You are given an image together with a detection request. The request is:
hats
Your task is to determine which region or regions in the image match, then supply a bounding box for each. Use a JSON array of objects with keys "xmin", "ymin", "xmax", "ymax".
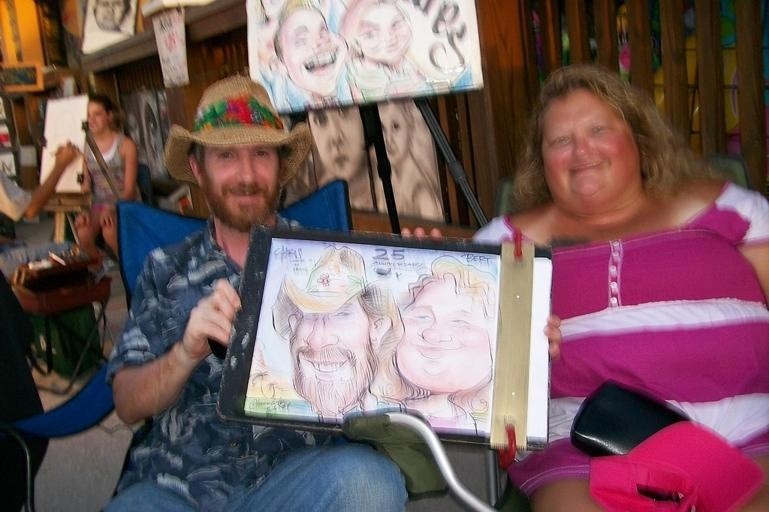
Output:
[{"xmin": 163, "ymin": 75, "xmax": 312, "ymax": 189}]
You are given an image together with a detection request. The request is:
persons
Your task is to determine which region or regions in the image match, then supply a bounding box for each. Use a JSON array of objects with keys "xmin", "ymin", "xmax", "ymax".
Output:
[
  {"xmin": 93, "ymin": 0, "xmax": 130, "ymax": 36},
  {"xmin": 97, "ymin": 72, "xmax": 562, "ymax": 512},
  {"xmin": 299, "ymin": 104, "xmax": 375, "ymax": 209},
  {"xmin": 391, "ymin": 253, "xmax": 495, "ymax": 429},
  {"xmin": 140, "ymin": 97, "xmax": 164, "ymax": 181},
  {"xmin": 270, "ymin": 244, "xmax": 406, "ymax": 419},
  {"xmin": 0, "ymin": 139, "xmax": 78, "ymax": 286},
  {"xmin": 336, "ymin": 0, "xmax": 428, "ymax": 80},
  {"xmin": 158, "ymin": 88, "xmax": 172, "ymax": 140},
  {"xmin": 74, "ymin": 92, "xmax": 139, "ymax": 262},
  {"xmin": 373, "ymin": 97, "xmax": 444, "ymax": 220},
  {"xmin": 398, "ymin": 63, "xmax": 768, "ymax": 511},
  {"xmin": 266, "ymin": 4, "xmax": 358, "ymax": 108},
  {"xmin": 121, "ymin": 99, "xmax": 146, "ymax": 164}
]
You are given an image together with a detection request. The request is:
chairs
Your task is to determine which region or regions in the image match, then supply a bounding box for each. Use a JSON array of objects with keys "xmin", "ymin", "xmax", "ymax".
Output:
[
  {"xmin": 96, "ymin": 163, "xmax": 157, "ymax": 266},
  {"xmin": 114, "ymin": 176, "xmax": 354, "ymax": 317}
]
